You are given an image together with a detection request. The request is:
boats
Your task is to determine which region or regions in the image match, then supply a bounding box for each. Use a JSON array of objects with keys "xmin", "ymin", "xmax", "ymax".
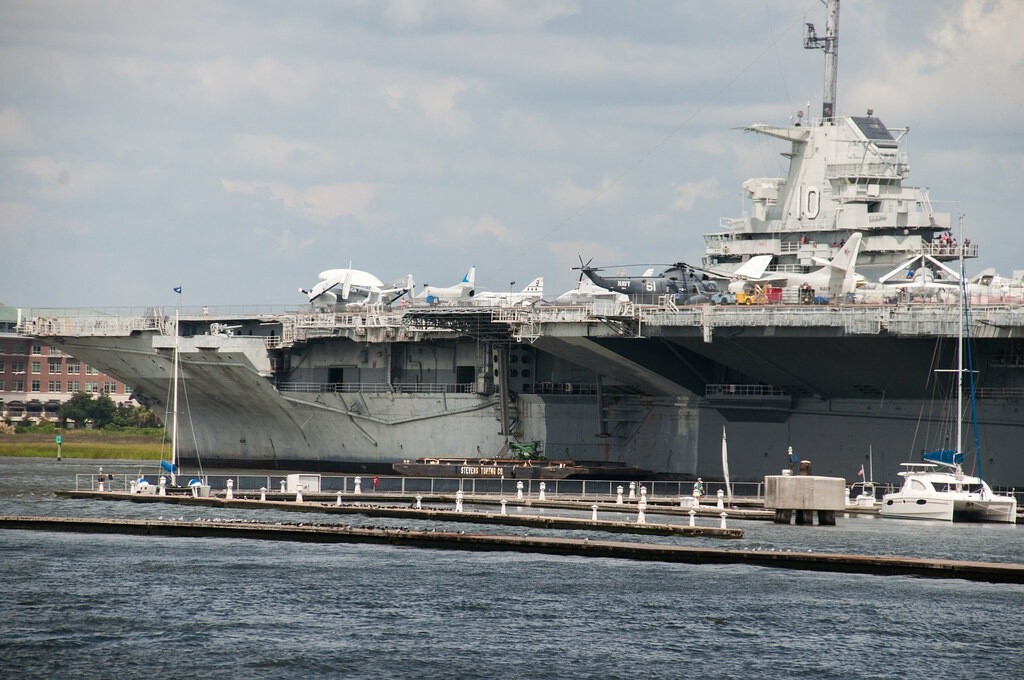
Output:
[{"xmin": 392, "ymin": 457, "xmax": 659, "ymax": 483}]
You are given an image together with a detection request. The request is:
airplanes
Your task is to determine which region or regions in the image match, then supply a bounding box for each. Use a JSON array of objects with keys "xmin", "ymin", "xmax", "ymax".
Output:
[{"xmin": 297, "ymin": 228, "xmax": 1023, "ymax": 313}]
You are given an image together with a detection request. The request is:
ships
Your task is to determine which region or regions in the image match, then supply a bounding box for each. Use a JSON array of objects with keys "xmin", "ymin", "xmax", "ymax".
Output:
[{"xmin": 11, "ymin": 2, "xmax": 1024, "ymax": 507}]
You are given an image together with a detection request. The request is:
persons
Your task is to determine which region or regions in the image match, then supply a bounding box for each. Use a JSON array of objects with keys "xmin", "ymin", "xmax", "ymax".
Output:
[
  {"xmin": 202, "ymin": 305, "xmax": 208, "ymax": 317},
  {"xmin": 935, "ymin": 231, "xmax": 957, "ymax": 254},
  {"xmin": 962, "ymin": 238, "xmax": 971, "ymax": 254},
  {"xmin": 400, "ymin": 296, "xmax": 411, "ymax": 311},
  {"xmin": 832, "ymin": 239, "xmax": 845, "ymax": 247}
]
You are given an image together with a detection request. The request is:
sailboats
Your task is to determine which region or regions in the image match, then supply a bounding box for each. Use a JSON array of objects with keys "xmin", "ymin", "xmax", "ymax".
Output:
[
  {"xmin": 879, "ymin": 211, "xmax": 1017, "ymax": 524},
  {"xmin": 129, "ymin": 307, "xmax": 221, "ymax": 496}
]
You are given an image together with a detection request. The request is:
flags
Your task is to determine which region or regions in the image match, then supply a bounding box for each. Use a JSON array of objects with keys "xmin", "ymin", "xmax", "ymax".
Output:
[{"xmin": 173, "ymin": 287, "xmax": 182, "ymax": 294}]
